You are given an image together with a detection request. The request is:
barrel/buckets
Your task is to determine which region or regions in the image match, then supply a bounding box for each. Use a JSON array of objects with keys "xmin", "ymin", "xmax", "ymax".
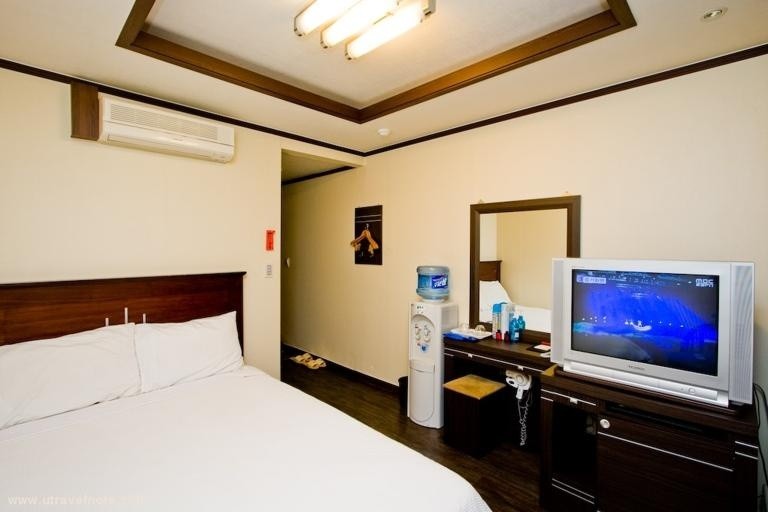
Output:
[{"xmin": 416, "ymin": 265, "xmax": 449, "ymax": 300}]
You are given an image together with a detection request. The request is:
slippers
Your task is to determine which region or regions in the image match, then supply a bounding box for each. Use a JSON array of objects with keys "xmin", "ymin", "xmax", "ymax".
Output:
[{"xmin": 290, "ymin": 353, "xmax": 326, "ymax": 369}]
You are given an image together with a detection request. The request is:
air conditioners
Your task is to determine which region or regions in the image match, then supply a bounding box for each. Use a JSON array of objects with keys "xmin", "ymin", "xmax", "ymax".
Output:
[{"xmin": 97, "ymin": 91, "xmax": 235, "ymax": 165}]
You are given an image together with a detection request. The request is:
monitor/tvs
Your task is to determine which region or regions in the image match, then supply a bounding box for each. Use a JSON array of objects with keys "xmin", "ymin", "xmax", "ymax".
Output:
[{"xmin": 563, "ymin": 257, "xmax": 731, "ymax": 408}]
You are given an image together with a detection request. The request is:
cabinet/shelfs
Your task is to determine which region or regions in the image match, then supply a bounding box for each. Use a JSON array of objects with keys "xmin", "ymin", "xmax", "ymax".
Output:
[{"xmin": 538, "ymin": 363, "xmax": 759, "ymax": 512}]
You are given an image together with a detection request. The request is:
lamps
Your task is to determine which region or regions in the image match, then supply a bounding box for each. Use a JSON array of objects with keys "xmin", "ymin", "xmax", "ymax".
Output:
[{"xmin": 293, "ymin": 0, "xmax": 436, "ymax": 61}]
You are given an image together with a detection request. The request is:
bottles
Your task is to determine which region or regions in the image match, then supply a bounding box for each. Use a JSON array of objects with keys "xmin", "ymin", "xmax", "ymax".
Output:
[
  {"xmin": 517, "ymin": 315, "xmax": 525, "ymax": 330},
  {"xmin": 491, "ymin": 303, "xmax": 520, "ymax": 345}
]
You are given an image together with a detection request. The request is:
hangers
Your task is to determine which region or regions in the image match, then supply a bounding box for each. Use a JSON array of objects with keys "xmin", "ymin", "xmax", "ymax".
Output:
[{"xmin": 350, "ymin": 224, "xmax": 379, "ymax": 255}]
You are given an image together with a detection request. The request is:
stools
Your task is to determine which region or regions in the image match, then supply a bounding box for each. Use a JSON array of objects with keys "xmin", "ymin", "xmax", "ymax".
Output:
[{"xmin": 441, "ymin": 374, "xmax": 508, "ymax": 459}]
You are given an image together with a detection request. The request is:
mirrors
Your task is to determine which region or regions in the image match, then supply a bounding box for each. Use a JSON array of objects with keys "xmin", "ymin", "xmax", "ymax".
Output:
[{"xmin": 468, "ymin": 195, "xmax": 581, "ymax": 344}]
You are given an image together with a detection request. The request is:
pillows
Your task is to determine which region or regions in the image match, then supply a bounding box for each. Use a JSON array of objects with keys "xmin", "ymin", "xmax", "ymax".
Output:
[
  {"xmin": 479, "ymin": 280, "xmax": 513, "ymax": 324},
  {"xmin": 0, "ymin": 321, "xmax": 142, "ymax": 430},
  {"xmin": 134, "ymin": 310, "xmax": 246, "ymax": 392}
]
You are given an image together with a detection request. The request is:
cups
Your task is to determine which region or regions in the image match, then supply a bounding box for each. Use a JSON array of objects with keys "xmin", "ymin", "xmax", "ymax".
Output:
[{"xmin": 474, "ymin": 325, "xmax": 486, "ymax": 337}]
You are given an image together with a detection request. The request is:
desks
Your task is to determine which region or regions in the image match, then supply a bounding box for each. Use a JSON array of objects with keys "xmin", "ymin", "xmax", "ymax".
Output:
[{"xmin": 443, "ymin": 328, "xmax": 555, "ymax": 454}]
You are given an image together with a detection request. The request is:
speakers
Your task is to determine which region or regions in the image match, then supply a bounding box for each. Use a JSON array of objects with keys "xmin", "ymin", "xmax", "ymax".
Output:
[
  {"xmin": 729, "ymin": 261, "xmax": 755, "ymax": 405},
  {"xmin": 550, "ymin": 257, "xmax": 564, "ymax": 365}
]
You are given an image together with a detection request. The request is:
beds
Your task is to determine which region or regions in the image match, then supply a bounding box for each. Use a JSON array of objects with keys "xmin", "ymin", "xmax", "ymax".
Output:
[
  {"xmin": 479, "ymin": 260, "xmax": 551, "ymax": 334},
  {"xmin": 0, "ymin": 270, "xmax": 496, "ymax": 512}
]
